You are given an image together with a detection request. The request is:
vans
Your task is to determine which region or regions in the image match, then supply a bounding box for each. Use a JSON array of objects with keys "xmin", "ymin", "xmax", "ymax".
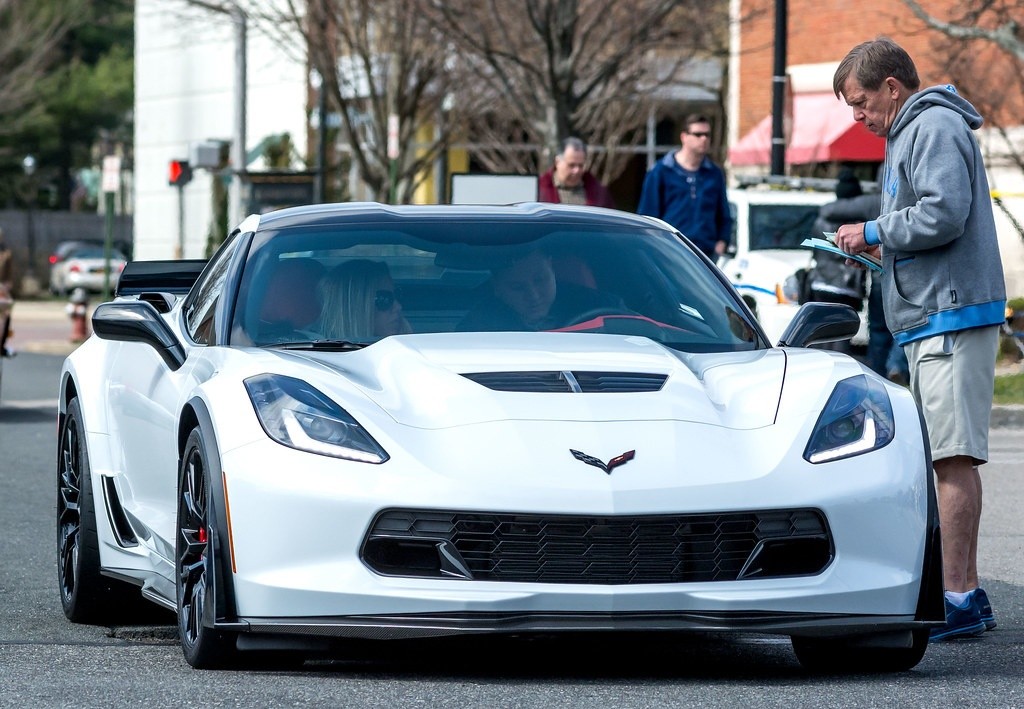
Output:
[{"xmin": 711, "ymin": 182, "xmax": 875, "ymax": 351}]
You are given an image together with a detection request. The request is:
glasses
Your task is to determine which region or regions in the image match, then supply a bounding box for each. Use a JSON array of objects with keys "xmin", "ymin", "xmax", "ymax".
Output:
[
  {"xmin": 688, "ymin": 132, "xmax": 711, "ymax": 137},
  {"xmin": 376, "ymin": 286, "xmax": 402, "ymax": 312}
]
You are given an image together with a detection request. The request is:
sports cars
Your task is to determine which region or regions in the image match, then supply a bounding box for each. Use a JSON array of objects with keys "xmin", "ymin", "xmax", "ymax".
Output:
[{"xmin": 54, "ymin": 200, "xmax": 950, "ymax": 675}]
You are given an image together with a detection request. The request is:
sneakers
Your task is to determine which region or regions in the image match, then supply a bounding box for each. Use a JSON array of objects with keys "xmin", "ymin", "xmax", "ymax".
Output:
[{"xmin": 929, "ymin": 588, "xmax": 997, "ymax": 642}]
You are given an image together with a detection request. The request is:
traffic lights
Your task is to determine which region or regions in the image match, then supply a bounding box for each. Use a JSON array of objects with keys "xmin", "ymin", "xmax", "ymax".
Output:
[{"xmin": 170, "ymin": 160, "xmax": 193, "ymax": 189}]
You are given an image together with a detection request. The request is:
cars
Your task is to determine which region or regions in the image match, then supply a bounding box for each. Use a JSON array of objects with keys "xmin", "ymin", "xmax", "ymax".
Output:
[{"xmin": 47, "ymin": 243, "xmax": 129, "ymax": 294}]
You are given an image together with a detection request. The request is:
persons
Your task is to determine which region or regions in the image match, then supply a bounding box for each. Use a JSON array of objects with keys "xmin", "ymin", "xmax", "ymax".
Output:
[
  {"xmin": 303, "ymin": 259, "xmax": 411, "ymax": 345},
  {"xmin": 832, "ymin": 40, "xmax": 1006, "ymax": 640},
  {"xmin": 0, "ymin": 242, "xmax": 17, "ymax": 355},
  {"xmin": 537, "ymin": 137, "xmax": 613, "ymax": 206},
  {"xmin": 639, "ymin": 114, "xmax": 730, "ymax": 261},
  {"xmin": 456, "ymin": 247, "xmax": 626, "ymax": 339}
]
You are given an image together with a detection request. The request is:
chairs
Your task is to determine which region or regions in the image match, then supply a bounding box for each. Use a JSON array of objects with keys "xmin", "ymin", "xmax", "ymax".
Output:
[{"xmin": 269, "ymin": 259, "xmax": 327, "ymax": 332}]
